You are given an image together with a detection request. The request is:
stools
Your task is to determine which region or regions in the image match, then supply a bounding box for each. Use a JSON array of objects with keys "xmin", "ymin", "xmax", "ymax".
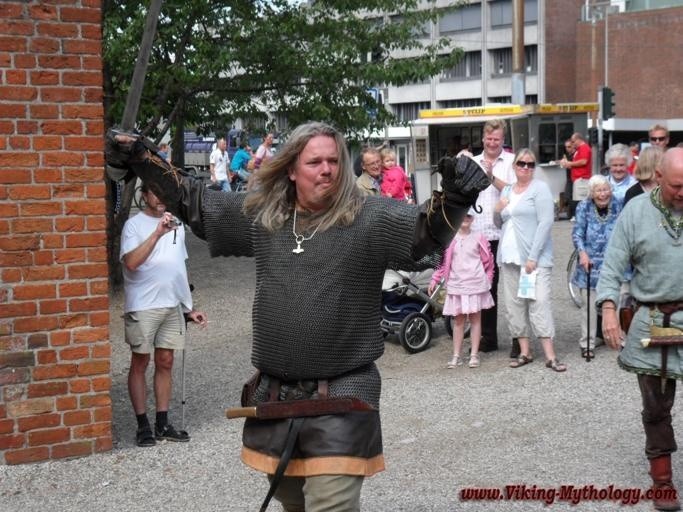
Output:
[{"xmin": 230, "ymin": 172, "xmax": 247, "ymax": 191}]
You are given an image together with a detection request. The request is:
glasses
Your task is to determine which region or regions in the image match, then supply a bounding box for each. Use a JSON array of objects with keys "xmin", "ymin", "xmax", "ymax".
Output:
[
  {"xmin": 649, "ymin": 136, "xmax": 667, "ymax": 142},
  {"xmin": 517, "ymin": 160, "xmax": 536, "ymax": 169}
]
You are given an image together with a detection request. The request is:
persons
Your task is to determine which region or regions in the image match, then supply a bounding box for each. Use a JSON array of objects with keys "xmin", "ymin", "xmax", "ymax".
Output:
[
  {"xmin": 560, "ymin": 124, "xmax": 683, "ymax": 361},
  {"xmin": 152, "ymin": 128, "xmax": 278, "ymax": 196},
  {"xmin": 596, "ymin": 148, "xmax": 683, "ymax": 512},
  {"xmin": 356, "ymin": 116, "xmax": 568, "ymax": 373},
  {"xmin": 118, "ymin": 180, "xmax": 205, "ymax": 449},
  {"xmin": 106, "ymin": 122, "xmax": 490, "ymax": 511}
]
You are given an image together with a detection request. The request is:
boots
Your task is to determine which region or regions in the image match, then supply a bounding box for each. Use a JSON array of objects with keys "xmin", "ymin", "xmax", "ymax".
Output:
[{"xmin": 649, "ymin": 454, "xmax": 680, "ymax": 511}]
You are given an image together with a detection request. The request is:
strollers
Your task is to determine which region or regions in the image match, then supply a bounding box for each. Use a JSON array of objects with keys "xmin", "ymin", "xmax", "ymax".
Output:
[{"xmin": 380, "ymin": 267, "xmax": 469, "ymax": 353}]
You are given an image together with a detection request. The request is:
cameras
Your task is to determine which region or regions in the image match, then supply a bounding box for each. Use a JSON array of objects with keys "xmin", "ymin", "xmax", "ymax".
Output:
[{"xmin": 168, "ymin": 216, "xmax": 183, "ymax": 227}]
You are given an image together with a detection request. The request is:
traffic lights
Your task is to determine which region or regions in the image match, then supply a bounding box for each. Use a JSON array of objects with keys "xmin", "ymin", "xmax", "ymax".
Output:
[{"xmin": 601, "ymin": 88, "xmax": 616, "ymax": 120}]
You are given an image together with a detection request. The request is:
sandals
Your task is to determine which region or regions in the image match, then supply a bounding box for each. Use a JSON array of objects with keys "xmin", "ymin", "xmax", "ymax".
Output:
[
  {"xmin": 448, "ymin": 343, "xmax": 595, "ymax": 372},
  {"xmin": 136, "ymin": 426, "xmax": 155, "ymax": 447},
  {"xmin": 154, "ymin": 423, "xmax": 190, "ymax": 442}
]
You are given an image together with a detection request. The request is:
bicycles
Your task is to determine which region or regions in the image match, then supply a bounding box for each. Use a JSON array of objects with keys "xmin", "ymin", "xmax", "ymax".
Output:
[{"xmin": 566, "ymin": 248, "xmax": 583, "ymax": 309}]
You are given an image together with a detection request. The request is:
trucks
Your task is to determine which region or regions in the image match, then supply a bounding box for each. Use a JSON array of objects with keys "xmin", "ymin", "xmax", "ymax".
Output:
[{"xmin": 183, "ymin": 130, "xmax": 242, "ymax": 180}]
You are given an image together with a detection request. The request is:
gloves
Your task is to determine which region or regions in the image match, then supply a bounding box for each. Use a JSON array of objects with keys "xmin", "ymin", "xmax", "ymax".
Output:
[
  {"xmin": 104, "ymin": 127, "xmax": 208, "ymax": 242},
  {"xmin": 410, "ymin": 154, "xmax": 491, "ymax": 264}
]
